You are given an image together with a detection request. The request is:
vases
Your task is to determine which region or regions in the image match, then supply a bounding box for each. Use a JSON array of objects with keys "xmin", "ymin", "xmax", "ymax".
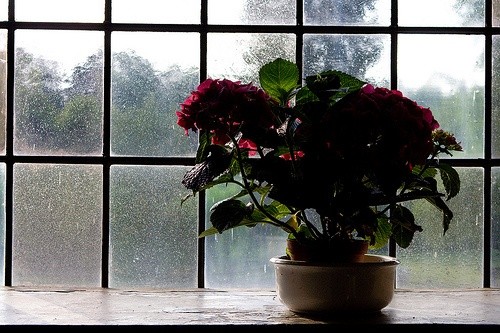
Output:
[{"xmin": 269, "ymin": 239, "xmax": 402, "ymax": 321}]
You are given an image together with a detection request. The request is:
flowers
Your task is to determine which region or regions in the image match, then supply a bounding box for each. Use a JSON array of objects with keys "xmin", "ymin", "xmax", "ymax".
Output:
[{"xmin": 174, "ymin": 56, "xmax": 465, "ymax": 239}]
